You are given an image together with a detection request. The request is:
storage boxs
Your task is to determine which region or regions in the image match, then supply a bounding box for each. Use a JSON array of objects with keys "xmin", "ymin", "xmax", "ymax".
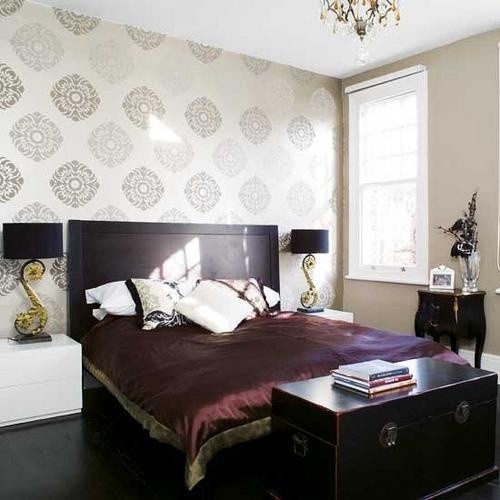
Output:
[{"xmin": 272, "ymin": 356, "xmax": 499, "ymax": 500}]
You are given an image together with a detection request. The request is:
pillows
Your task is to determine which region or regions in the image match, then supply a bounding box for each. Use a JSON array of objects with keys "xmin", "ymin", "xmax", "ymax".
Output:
[
  {"xmin": 178, "ymin": 282, "xmax": 255, "ymax": 335},
  {"xmin": 84, "ymin": 278, "xmax": 279, "ymax": 321},
  {"xmin": 126, "ymin": 277, "xmax": 194, "ymax": 332},
  {"xmin": 203, "ymin": 278, "xmax": 268, "ymax": 322}
]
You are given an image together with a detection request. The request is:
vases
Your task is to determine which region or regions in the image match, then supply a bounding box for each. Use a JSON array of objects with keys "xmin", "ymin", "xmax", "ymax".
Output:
[{"xmin": 455, "ymin": 250, "xmax": 480, "ymax": 294}]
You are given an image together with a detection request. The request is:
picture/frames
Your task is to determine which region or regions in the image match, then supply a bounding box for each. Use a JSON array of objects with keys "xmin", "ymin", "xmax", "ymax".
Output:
[{"xmin": 430, "ymin": 266, "xmax": 454, "ymax": 289}]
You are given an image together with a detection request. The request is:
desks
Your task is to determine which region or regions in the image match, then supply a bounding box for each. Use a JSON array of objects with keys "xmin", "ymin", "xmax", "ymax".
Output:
[{"xmin": 414, "ymin": 288, "xmax": 487, "ymax": 368}]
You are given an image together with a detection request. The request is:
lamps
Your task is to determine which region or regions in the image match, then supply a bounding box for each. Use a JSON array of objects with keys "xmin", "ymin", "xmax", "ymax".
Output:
[
  {"xmin": 291, "ymin": 230, "xmax": 330, "ymax": 312},
  {"xmin": 321, "ymin": 0, "xmax": 399, "ymax": 42},
  {"xmin": 4, "ymin": 224, "xmax": 62, "ymax": 344}
]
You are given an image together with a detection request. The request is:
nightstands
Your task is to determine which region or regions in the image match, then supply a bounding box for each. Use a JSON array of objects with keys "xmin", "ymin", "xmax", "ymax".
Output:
[
  {"xmin": 292, "ymin": 309, "xmax": 354, "ymax": 324},
  {"xmin": 0, "ymin": 336, "xmax": 82, "ymax": 431}
]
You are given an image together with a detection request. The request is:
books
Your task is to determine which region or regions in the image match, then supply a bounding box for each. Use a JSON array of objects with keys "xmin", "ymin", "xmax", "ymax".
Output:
[{"xmin": 330, "ymin": 357, "xmax": 417, "ymax": 402}]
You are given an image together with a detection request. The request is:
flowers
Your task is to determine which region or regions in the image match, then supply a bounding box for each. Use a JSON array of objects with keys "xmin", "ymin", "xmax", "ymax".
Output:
[{"xmin": 437, "ymin": 190, "xmax": 480, "ymax": 279}]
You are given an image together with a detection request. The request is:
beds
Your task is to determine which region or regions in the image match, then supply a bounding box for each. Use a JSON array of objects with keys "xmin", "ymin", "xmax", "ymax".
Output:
[{"xmin": 68, "ymin": 220, "xmax": 474, "ymax": 500}]
